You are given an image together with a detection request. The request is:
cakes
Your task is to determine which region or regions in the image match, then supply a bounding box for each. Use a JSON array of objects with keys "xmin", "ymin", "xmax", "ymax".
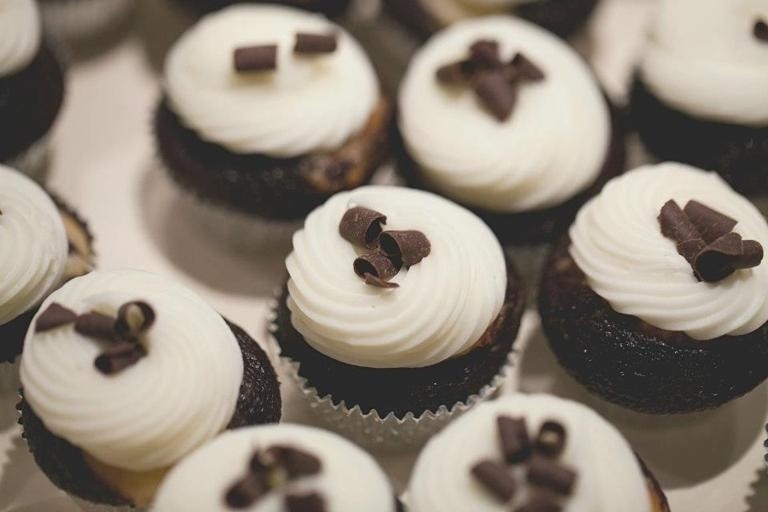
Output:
[{"xmin": 0, "ymin": 0, "xmax": 768, "ymax": 512}]
